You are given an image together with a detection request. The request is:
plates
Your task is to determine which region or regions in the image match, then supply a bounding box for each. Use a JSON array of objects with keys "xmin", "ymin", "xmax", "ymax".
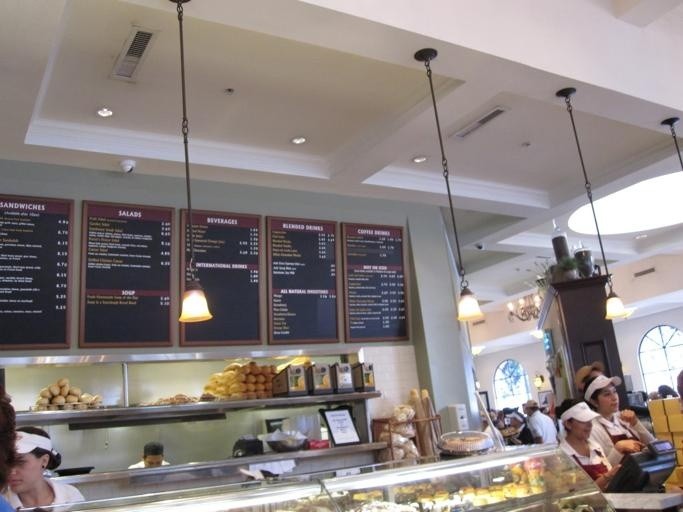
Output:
[{"xmin": 53, "ymin": 466, "xmax": 95, "ymax": 477}]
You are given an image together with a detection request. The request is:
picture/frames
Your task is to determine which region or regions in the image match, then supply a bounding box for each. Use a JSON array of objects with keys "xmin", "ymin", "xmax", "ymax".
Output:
[
  {"xmin": 318, "ymin": 404, "xmax": 361, "ymax": 446},
  {"xmin": 478, "ymin": 391, "xmax": 490, "ymax": 413}
]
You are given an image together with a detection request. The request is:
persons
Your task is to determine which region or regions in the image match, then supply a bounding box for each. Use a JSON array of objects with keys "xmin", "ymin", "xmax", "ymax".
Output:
[
  {"xmin": 482, "ymin": 360, "xmax": 683, "ymax": 493},
  {"xmin": 0, "ymin": 384, "xmax": 19, "ymax": 512},
  {"xmin": 2, "ymin": 426, "xmax": 85, "ymax": 512},
  {"xmin": 126, "ymin": 440, "xmax": 170, "ymax": 487}
]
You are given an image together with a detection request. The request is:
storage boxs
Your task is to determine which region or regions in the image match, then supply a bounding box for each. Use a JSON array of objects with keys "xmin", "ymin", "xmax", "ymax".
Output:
[
  {"xmin": 353, "ymin": 362, "xmax": 376, "ymax": 392},
  {"xmin": 330, "ymin": 362, "xmax": 355, "ymax": 395},
  {"xmin": 306, "ymin": 362, "xmax": 334, "ymax": 395},
  {"xmin": 272, "ymin": 364, "xmax": 308, "ymax": 397}
]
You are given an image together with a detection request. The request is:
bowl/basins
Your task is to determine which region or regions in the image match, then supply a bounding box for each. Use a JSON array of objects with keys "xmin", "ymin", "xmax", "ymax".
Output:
[{"xmin": 265, "ymin": 438, "xmax": 308, "ymax": 451}]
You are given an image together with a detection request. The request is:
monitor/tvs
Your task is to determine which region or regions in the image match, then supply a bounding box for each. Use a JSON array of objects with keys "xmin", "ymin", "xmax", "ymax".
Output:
[{"xmin": 606, "ymin": 447, "xmax": 679, "ymax": 492}]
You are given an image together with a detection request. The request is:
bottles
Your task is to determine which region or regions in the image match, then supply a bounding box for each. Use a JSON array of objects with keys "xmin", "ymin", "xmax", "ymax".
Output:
[{"xmin": 550, "ymin": 217, "xmax": 594, "ymax": 277}]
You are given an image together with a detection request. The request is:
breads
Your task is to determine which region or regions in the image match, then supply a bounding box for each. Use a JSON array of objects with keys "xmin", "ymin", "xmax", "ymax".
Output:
[
  {"xmin": 140, "ymin": 361, "xmax": 277, "ymax": 406},
  {"xmin": 34, "ymin": 378, "xmax": 100, "ymax": 411},
  {"xmin": 417, "ymin": 481, "xmax": 529, "ymax": 511},
  {"xmin": 352, "ymin": 483, "xmax": 431, "ymax": 502},
  {"xmin": 377, "ymin": 389, "xmax": 429, "ymax": 462}
]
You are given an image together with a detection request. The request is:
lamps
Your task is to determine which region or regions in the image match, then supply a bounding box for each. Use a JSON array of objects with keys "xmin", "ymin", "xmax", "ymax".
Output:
[
  {"xmin": 533, "ymin": 373, "xmax": 545, "ymax": 388},
  {"xmin": 555, "ymin": 87, "xmax": 629, "ymax": 320},
  {"xmin": 168, "ymin": 0, "xmax": 214, "ymax": 324},
  {"xmin": 506, "ymin": 294, "xmax": 545, "ymax": 322},
  {"xmin": 413, "ymin": 48, "xmax": 486, "ymax": 322}
]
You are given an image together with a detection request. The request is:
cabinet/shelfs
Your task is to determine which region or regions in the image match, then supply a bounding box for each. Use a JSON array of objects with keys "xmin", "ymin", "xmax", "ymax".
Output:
[{"xmin": 15, "ymin": 389, "xmax": 388, "ymax": 485}]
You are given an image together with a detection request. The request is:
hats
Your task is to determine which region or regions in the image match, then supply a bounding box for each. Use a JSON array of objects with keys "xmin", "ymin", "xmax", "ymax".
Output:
[
  {"xmin": 13, "ymin": 429, "xmax": 58, "ymax": 458},
  {"xmin": 525, "ymin": 399, "xmax": 540, "ymax": 409},
  {"xmin": 573, "ymin": 360, "xmax": 606, "ymax": 392},
  {"xmin": 560, "ymin": 399, "xmax": 603, "ymax": 423},
  {"xmin": 582, "ymin": 374, "xmax": 624, "ymax": 403}
]
